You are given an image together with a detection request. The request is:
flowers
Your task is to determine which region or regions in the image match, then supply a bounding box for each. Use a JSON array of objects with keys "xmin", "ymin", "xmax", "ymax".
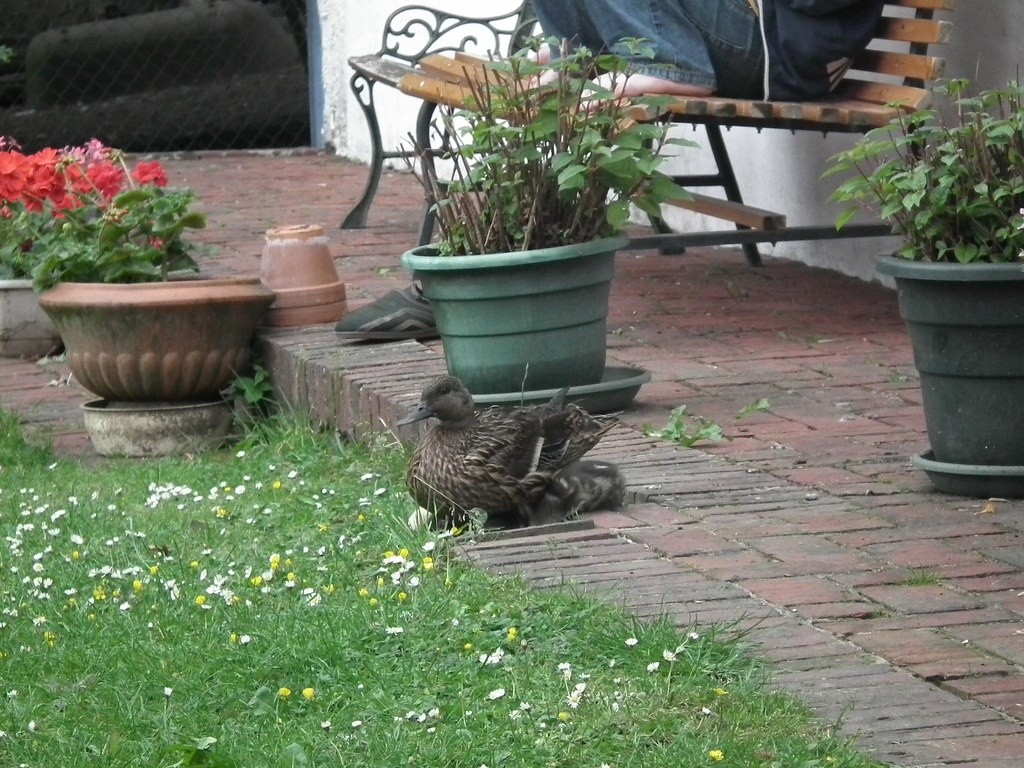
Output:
[{"xmin": 0, "ymin": 134, "xmax": 220, "ymax": 294}]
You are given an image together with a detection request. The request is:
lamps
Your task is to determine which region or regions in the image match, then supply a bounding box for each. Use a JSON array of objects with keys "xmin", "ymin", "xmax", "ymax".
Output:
[{"xmin": 0, "ymin": 279, "xmax": 64, "ymax": 358}]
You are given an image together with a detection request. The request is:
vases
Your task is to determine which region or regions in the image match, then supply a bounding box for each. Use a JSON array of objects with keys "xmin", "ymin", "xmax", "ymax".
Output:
[
  {"xmin": 44, "ymin": 275, "xmax": 278, "ymax": 407},
  {"xmin": 257, "ymin": 220, "xmax": 347, "ymax": 324}
]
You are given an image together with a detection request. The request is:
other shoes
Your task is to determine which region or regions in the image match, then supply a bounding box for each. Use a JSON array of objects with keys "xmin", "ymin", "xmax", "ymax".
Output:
[{"xmin": 335, "ymin": 284, "xmax": 441, "ymax": 339}]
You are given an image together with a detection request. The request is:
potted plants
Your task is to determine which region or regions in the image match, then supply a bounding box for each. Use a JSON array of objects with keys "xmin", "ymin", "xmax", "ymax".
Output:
[
  {"xmin": 822, "ymin": 53, "xmax": 1024, "ymax": 466},
  {"xmin": 398, "ymin": 33, "xmax": 702, "ymax": 394}
]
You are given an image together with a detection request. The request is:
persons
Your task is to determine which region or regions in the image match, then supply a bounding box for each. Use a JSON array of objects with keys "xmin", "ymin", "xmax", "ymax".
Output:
[{"xmin": 524, "ymin": 0, "xmax": 884, "ymax": 110}]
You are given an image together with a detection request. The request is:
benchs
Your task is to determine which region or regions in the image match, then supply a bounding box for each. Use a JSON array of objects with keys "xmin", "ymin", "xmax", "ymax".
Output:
[{"xmin": 341, "ymin": 0, "xmax": 959, "ymax": 283}]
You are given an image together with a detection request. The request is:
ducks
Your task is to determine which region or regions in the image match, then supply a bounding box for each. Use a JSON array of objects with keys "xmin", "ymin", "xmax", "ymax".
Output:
[{"xmin": 397, "ymin": 373, "xmax": 628, "ymax": 521}]
